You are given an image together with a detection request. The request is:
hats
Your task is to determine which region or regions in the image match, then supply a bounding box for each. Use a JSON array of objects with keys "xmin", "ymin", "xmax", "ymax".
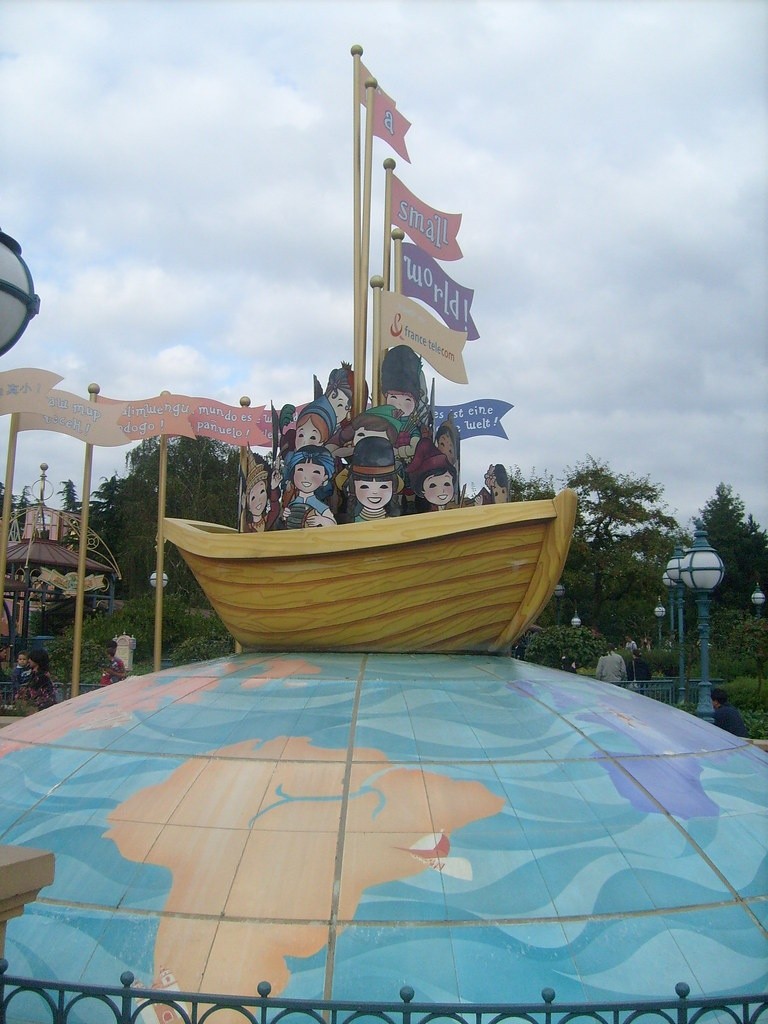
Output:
[
  {"xmin": 633, "ymin": 649, "xmax": 642, "ymax": 657},
  {"xmin": 107, "ymin": 647, "xmax": 115, "ymax": 657},
  {"xmin": 0, "ymin": 646, "xmax": 4, "ymax": 652},
  {"xmin": 28, "ymin": 648, "xmax": 50, "ymax": 667}
]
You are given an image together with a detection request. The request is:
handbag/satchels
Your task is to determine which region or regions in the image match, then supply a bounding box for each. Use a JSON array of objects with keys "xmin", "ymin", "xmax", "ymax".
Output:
[{"xmin": 628, "ymin": 680, "xmax": 638, "ymax": 691}]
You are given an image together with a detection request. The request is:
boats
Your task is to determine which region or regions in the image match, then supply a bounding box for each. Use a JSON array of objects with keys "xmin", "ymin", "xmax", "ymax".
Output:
[{"xmin": 159, "ymin": 42, "xmax": 581, "ymax": 653}]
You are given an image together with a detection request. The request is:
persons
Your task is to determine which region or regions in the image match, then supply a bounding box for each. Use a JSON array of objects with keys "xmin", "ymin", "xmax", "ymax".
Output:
[
  {"xmin": 711, "ymin": 688, "xmax": 749, "ymax": 738},
  {"xmin": 98, "ymin": 640, "xmax": 127, "ymax": 685},
  {"xmin": 596, "ymin": 631, "xmax": 672, "ymax": 695},
  {"xmin": 0, "ymin": 647, "xmax": 57, "ymax": 711}
]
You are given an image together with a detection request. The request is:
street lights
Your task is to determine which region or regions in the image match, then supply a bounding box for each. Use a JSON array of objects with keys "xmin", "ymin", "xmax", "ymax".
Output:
[
  {"xmin": 653, "ymin": 519, "xmax": 727, "ymax": 726},
  {"xmin": 554, "ymin": 584, "xmax": 581, "ymax": 629},
  {"xmin": 750, "ymin": 582, "xmax": 766, "ymax": 619}
]
